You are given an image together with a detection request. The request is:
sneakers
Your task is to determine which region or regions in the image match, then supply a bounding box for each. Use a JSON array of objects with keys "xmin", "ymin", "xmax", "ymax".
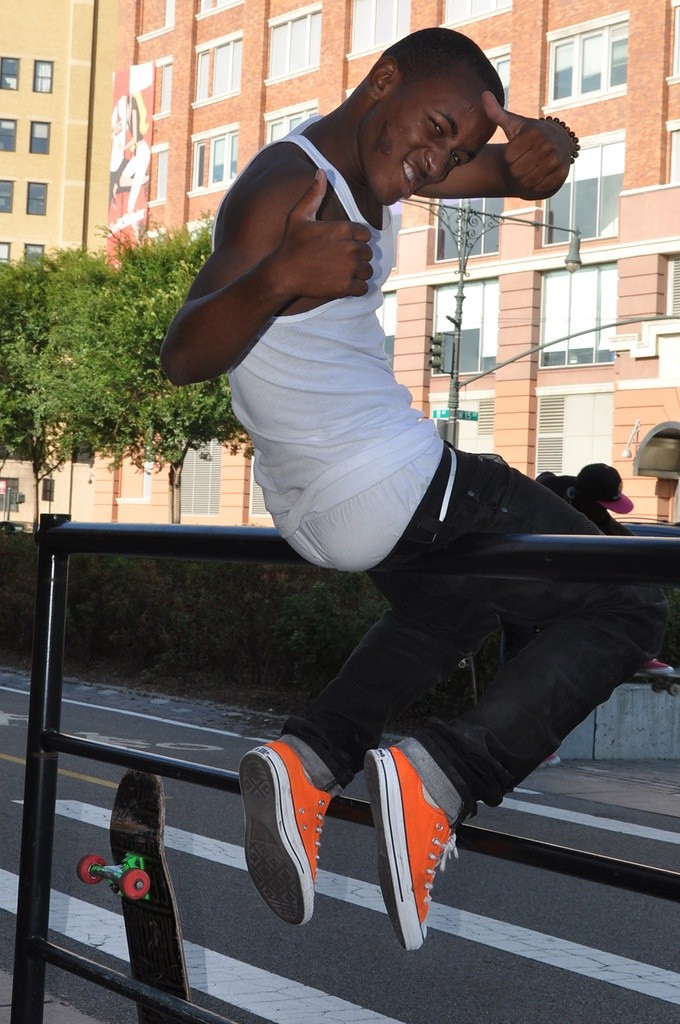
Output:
[
  {"xmin": 239, "ymin": 740, "xmax": 331, "ymax": 926},
  {"xmin": 636, "ymin": 658, "xmax": 675, "ymax": 674},
  {"xmin": 366, "ymin": 745, "xmax": 456, "ymax": 951}
]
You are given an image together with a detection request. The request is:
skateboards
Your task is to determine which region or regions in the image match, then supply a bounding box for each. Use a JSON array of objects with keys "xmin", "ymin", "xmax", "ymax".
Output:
[{"xmin": 73, "ymin": 768, "xmax": 194, "ymax": 1024}]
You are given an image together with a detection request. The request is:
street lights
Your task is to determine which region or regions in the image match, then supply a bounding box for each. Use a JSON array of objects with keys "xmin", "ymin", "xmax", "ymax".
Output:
[{"xmin": 399, "ymin": 194, "xmax": 582, "ymax": 450}]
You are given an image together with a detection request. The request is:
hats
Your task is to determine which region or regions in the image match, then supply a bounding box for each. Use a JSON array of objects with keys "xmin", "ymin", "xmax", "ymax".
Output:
[{"xmin": 578, "ymin": 463, "xmax": 633, "ymax": 514}]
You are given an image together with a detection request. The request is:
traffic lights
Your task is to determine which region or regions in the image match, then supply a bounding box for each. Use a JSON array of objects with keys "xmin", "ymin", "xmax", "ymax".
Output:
[{"xmin": 428, "ymin": 333, "xmax": 445, "ymax": 370}]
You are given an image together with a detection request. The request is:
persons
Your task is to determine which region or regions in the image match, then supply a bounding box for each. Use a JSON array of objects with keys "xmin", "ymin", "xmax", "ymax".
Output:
[
  {"xmin": 534, "ymin": 464, "xmax": 674, "ymax": 675},
  {"xmin": 159, "ymin": 27, "xmax": 668, "ymax": 956}
]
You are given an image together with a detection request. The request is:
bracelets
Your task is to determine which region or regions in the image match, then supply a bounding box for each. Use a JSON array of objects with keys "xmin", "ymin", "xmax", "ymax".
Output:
[{"xmin": 539, "ymin": 116, "xmax": 582, "ymax": 164}]
